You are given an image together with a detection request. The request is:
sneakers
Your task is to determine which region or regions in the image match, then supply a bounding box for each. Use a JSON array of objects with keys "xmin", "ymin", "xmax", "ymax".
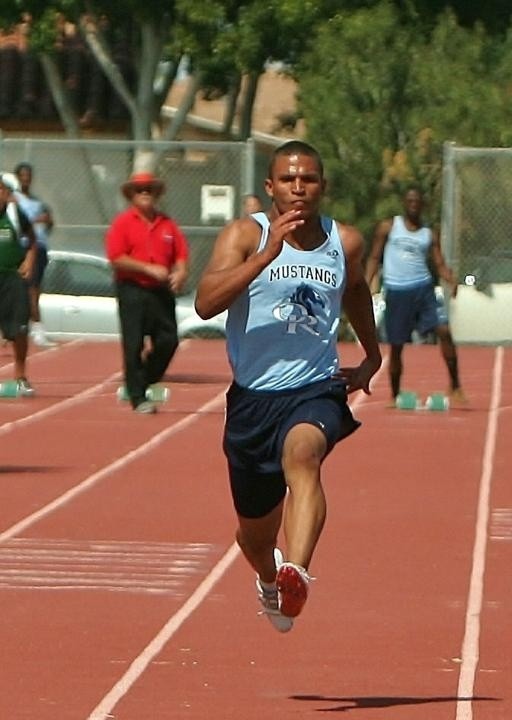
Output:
[
  {"xmin": 448, "ymin": 387, "xmax": 465, "ymax": 402},
  {"xmin": 16, "ymin": 377, "xmax": 34, "ymax": 394},
  {"xmin": 135, "ymin": 402, "xmax": 156, "ymax": 414},
  {"xmin": 255, "ymin": 548, "xmax": 316, "ymax": 634}
]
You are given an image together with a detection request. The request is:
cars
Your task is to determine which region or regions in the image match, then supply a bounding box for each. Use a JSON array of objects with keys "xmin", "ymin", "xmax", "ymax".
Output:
[{"xmin": 28, "ymin": 250, "xmax": 228, "ymax": 337}]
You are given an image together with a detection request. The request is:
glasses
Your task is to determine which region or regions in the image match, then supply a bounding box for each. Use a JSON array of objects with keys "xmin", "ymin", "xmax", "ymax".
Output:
[{"xmin": 135, "ymin": 186, "xmax": 152, "ymax": 193}]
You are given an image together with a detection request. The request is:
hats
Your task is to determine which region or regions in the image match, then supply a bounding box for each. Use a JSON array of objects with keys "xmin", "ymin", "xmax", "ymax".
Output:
[{"xmin": 121, "ymin": 172, "xmax": 162, "ymax": 197}]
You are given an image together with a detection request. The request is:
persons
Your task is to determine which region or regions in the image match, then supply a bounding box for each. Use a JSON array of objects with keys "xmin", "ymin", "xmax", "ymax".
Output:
[
  {"xmin": 194, "ymin": 143, "xmax": 383, "ymax": 633},
  {"xmin": 242, "ymin": 195, "xmax": 262, "ymax": 213},
  {"xmin": 105, "ymin": 174, "xmax": 189, "ymax": 415},
  {"xmin": 0, "ymin": 171, "xmax": 38, "ymax": 396},
  {"xmin": 14, "ymin": 163, "xmax": 59, "ymax": 348},
  {"xmin": 364, "ymin": 188, "xmax": 472, "ymax": 411}
]
